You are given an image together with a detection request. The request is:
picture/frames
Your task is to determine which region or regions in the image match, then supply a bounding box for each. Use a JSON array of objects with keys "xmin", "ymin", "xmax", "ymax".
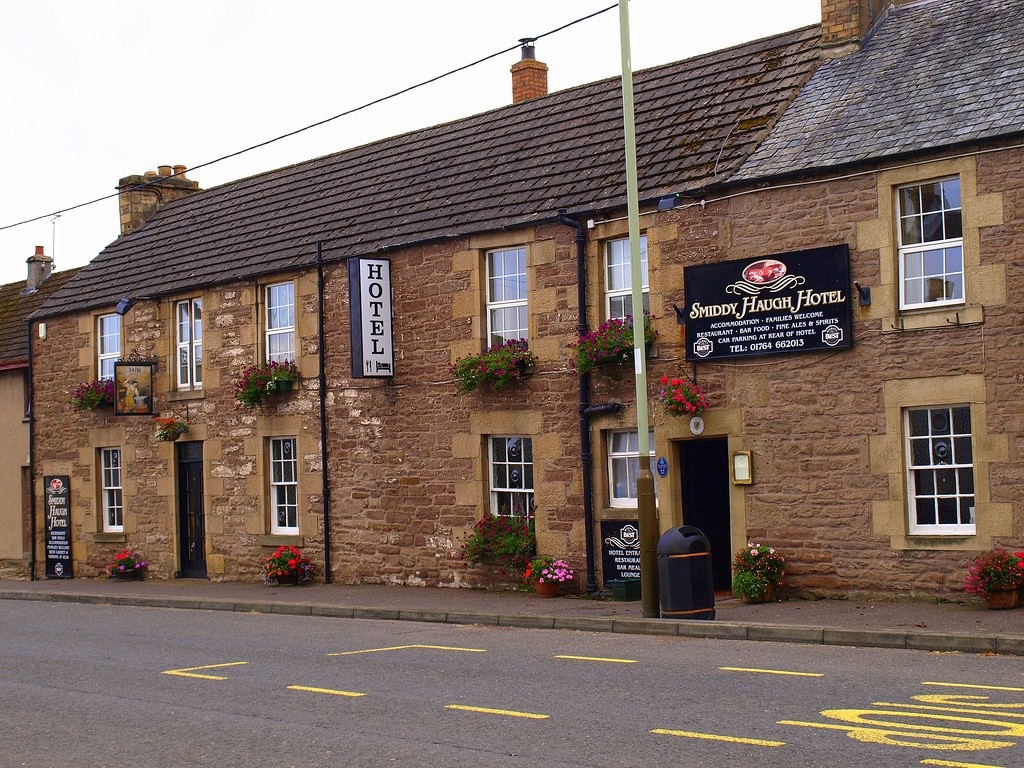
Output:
[
  {"xmin": 986, "ymin": 582, "xmax": 1021, "ymax": 610},
  {"xmin": 740, "ymin": 578, "xmax": 776, "ymax": 602},
  {"xmin": 113, "ymin": 361, "xmax": 156, "ymax": 417},
  {"xmin": 116, "ymin": 570, "xmax": 139, "ymax": 579}
]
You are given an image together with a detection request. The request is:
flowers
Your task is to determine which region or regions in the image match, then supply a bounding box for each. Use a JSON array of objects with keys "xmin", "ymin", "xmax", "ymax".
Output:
[
  {"xmin": 231, "ymin": 358, "xmax": 303, "ymax": 416},
  {"xmin": 448, "ymin": 337, "xmax": 539, "ymax": 395},
  {"xmin": 71, "ymin": 376, "xmax": 113, "ymax": 412},
  {"xmin": 106, "ymin": 549, "xmax": 147, "ymax": 576},
  {"xmin": 964, "ymin": 547, "xmax": 1024, "ymax": 604},
  {"xmin": 263, "ymin": 545, "xmax": 314, "ymax": 584},
  {"xmin": 455, "ymin": 503, "xmax": 538, "ymax": 578},
  {"xmin": 568, "ymin": 296, "xmax": 659, "ymax": 379},
  {"xmin": 732, "ymin": 542, "xmax": 790, "ymax": 603},
  {"xmin": 524, "ymin": 554, "xmax": 576, "ymax": 585},
  {"xmin": 659, "ymin": 366, "xmax": 710, "ymax": 416},
  {"xmin": 143, "ymin": 416, "xmax": 188, "ymax": 442}
]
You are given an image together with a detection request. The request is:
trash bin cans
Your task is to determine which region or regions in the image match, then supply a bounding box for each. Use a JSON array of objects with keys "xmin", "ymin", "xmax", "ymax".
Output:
[{"xmin": 657, "ymin": 524, "xmax": 716, "ymax": 620}]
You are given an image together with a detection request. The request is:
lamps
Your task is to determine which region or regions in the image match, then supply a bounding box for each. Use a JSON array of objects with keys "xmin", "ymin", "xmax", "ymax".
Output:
[
  {"xmin": 114, "ymin": 297, "xmax": 154, "ymax": 315},
  {"xmin": 657, "ymin": 195, "xmax": 705, "ymax": 213}
]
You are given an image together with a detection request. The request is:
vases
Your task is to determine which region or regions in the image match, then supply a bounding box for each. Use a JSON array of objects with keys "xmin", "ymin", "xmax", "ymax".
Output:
[
  {"xmin": 274, "ymin": 574, "xmax": 297, "ymax": 586},
  {"xmin": 165, "ymin": 433, "xmax": 181, "ymax": 442},
  {"xmin": 275, "ymin": 379, "xmax": 292, "ymax": 393},
  {"xmin": 533, "ymin": 577, "xmax": 566, "ymax": 598}
]
